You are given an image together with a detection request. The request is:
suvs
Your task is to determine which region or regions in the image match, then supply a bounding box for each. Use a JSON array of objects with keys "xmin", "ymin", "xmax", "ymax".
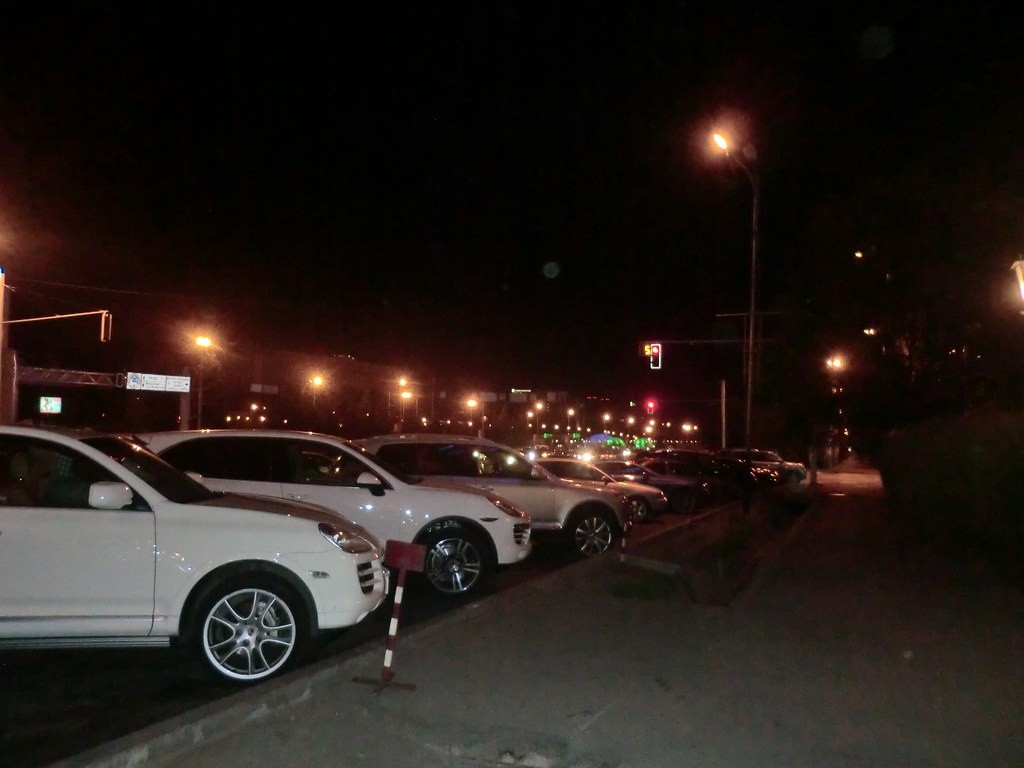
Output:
[
  {"xmin": 342, "ymin": 431, "xmax": 635, "ymax": 557},
  {"xmin": 1, "ymin": 418, "xmax": 398, "ymax": 687},
  {"xmin": 110, "ymin": 421, "xmax": 538, "ymax": 595}
]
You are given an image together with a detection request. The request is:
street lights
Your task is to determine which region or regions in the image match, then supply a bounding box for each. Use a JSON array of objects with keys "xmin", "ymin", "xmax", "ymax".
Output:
[
  {"xmin": 710, "ymin": 130, "xmax": 761, "ymax": 506},
  {"xmin": 177, "ymin": 322, "xmax": 695, "ymax": 460}
]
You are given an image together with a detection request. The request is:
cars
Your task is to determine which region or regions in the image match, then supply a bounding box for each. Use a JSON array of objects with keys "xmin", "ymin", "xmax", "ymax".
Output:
[{"xmin": 530, "ymin": 446, "xmax": 808, "ymax": 524}]
